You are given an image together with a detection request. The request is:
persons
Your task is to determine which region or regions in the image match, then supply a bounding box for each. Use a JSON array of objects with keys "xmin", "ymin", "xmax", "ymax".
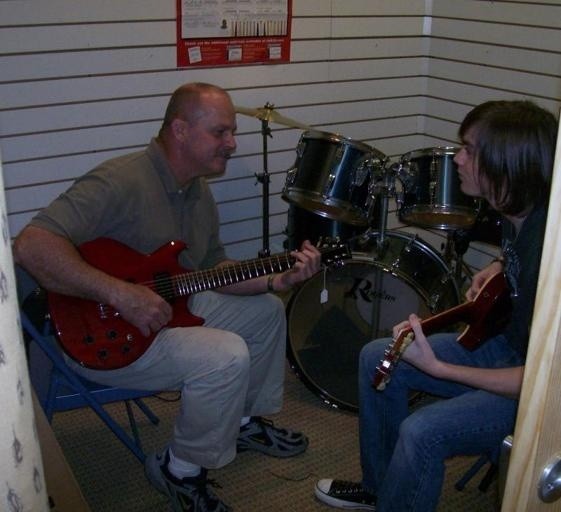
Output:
[
  {"xmin": 312, "ymin": 98, "xmax": 558, "ymax": 512},
  {"xmin": 9, "ymin": 81, "xmax": 310, "ymax": 512}
]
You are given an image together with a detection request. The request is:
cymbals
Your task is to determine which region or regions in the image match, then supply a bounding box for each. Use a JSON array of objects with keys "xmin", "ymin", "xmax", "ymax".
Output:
[{"xmin": 235, "ymin": 107, "xmax": 312, "ymax": 129}]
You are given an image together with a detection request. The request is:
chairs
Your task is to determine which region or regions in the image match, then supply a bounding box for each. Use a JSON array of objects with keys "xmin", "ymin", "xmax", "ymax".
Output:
[
  {"xmin": 9, "ymin": 263, "xmax": 171, "ymax": 467},
  {"xmin": 455, "ymin": 450, "xmax": 499, "ymax": 499}
]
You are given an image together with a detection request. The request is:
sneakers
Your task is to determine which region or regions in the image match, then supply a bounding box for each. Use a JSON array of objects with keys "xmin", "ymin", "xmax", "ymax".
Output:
[
  {"xmin": 145, "ymin": 446, "xmax": 230, "ymax": 512},
  {"xmin": 235, "ymin": 414, "xmax": 309, "ymax": 457},
  {"xmin": 312, "ymin": 478, "xmax": 376, "ymax": 509}
]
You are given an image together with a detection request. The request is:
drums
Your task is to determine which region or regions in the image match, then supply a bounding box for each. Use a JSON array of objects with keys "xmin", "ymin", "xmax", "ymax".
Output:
[
  {"xmin": 282, "ymin": 197, "xmax": 386, "ymax": 251},
  {"xmin": 285, "ymin": 227, "xmax": 461, "ymax": 413},
  {"xmin": 390, "ymin": 148, "xmax": 485, "ymax": 229},
  {"xmin": 281, "ymin": 130, "xmax": 389, "ymax": 227}
]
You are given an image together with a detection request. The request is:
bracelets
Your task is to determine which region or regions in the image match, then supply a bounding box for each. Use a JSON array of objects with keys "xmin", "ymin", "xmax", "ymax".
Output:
[{"xmin": 267, "ymin": 274, "xmax": 276, "ymax": 296}]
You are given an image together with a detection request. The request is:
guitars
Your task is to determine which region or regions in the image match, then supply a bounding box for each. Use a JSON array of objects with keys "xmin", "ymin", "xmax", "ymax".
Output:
[
  {"xmin": 48, "ymin": 237, "xmax": 352, "ymax": 371},
  {"xmin": 373, "ymin": 270, "xmax": 512, "ymax": 392}
]
